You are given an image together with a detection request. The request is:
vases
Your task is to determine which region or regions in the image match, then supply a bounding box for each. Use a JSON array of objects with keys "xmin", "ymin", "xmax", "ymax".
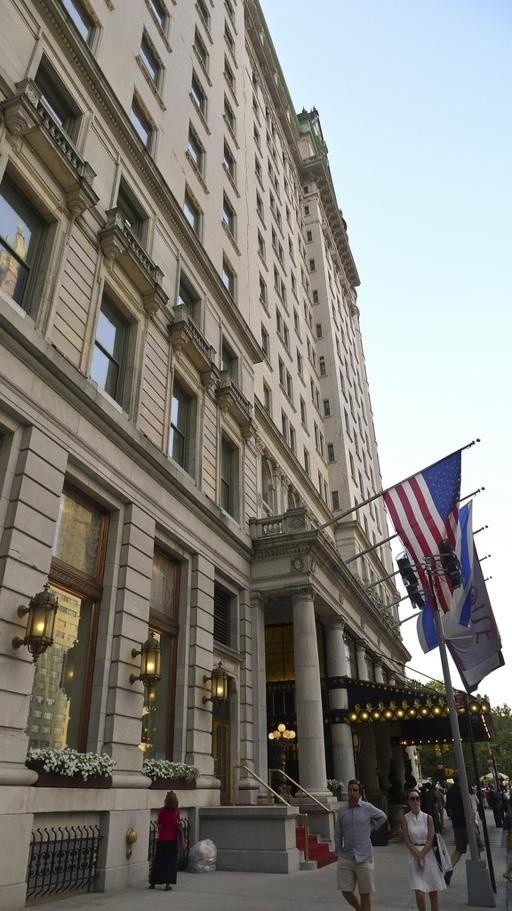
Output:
[
  {"xmin": 30, "ymin": 764, "xmax": 113, "ymax": 788},
  {"xmin": 146, "ymin": 774, "xmax": 196, "ymax": 790}
]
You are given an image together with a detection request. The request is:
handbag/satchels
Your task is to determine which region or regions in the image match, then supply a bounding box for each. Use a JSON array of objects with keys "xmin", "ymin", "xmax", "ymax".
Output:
[
  {"xmin": 433, "ymin": 833, "xmax": 453, "ymax": 872},
  {"xmin": 177, "ymin": 821, "xmax": 188, "ymax": 850}
]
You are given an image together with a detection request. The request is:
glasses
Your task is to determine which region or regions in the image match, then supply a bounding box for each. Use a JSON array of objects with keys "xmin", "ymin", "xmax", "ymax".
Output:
[{"xmin": 408, "ymin": 797, "xmax": 421, "ymax": 801}]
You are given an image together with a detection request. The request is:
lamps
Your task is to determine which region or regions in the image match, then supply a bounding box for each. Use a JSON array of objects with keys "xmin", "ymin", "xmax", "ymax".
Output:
[
  {"xmin": 12, "ymin": 583, "xmax": 58, "ymax": 662},
  {"xmin": 203, "ymin": 662, "xmax": 227, "ymax": 707},
  {"xmin": 129, "ymin": 632, "xmax": 160, "ymax": 685}
]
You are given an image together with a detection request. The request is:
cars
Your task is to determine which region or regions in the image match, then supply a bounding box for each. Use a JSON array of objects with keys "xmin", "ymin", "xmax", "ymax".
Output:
[{"xmin": 475, "ymin": 788, "xmax": 510, "ymax": 808}]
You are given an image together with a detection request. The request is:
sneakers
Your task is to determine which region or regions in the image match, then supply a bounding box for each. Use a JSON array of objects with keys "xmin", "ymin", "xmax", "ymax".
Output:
[{"xmin": 444, "ymin": 871, "xmax": 453, "ymax": 885}]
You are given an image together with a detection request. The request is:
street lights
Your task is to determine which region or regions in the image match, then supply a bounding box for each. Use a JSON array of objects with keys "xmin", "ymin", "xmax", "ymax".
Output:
[{"xmin": 268, "ymin": 722, "xmax": 296, "ymax": 803}]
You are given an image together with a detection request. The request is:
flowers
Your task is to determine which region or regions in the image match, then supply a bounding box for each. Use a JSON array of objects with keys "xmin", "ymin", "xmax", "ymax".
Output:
[
  {"xmin": 143, "ymin": 759, "xmax": 200, "ymax": 783},
  {"xmin": 26, "ymin": 745, "xmax": 114, "ymax": 779},
  {"xmin": 326, "ymin": 779, "xmax": 343, "ymax": 792}
]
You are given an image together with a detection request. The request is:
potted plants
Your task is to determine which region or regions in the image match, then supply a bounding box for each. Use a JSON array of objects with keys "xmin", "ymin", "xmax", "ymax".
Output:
[{"xmin": 387, "ymin": 786, "xmax": 408, "ymax": 834}]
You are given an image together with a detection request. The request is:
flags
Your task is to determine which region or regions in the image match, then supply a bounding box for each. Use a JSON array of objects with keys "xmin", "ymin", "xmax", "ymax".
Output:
[
  {"xmin": 381, "ymin": 453, "xmax": 462, "ymax": 619},
  {"xmin": 453, "ymin": 649, "xmax": 506, "ymax": 696},
  {"xmin": 416, "ymin": 497, "xmax": 475, "ymax": 652},
  {"xmin": 443, "ymin": 551, "xmax": 504, "ymax": 670}
]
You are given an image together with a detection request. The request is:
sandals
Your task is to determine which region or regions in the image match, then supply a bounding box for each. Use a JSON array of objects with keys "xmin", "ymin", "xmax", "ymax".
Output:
[
  {"xmin": 149, "ymin": 885, "xmax": 155, "ymax": 889},
  {"xmin": 164, "ymin": 885, "xmax": 172, "ymax": 890}
]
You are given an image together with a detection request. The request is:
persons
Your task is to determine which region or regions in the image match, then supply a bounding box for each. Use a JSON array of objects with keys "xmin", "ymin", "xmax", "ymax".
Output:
[
  {"xmin": 400, "ymin": 769, "xmax": 512, "ymax": 886},
  {"xmin": 334, "ymin": 778, "xmax": 388, "ymax": 911},
  {"xmin": 400, "ymin": 789, "xmax": 448, "ymax": 910},
  {"xmin": 147, "ymin": 791, "xmax": 186, "ymax": 891}
]
